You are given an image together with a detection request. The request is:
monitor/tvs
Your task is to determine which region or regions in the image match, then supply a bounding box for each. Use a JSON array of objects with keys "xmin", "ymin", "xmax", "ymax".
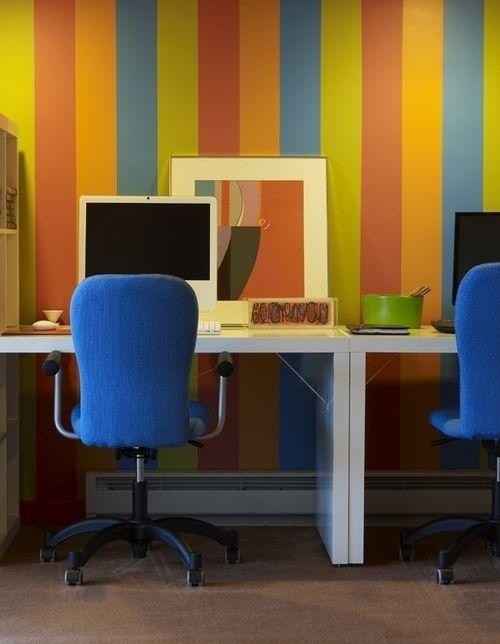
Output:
[
  {"xmin": 453, "ymin": 211, "xmax": 499, "ymax": 306},
  {"xmin": 78, "ymin": 196, "xmax": 217, "ymax": 312}
]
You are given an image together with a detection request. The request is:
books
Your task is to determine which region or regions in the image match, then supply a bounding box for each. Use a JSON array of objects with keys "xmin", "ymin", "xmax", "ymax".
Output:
[
  {"xmin": 343, "ymin": 319, "xmax": 412, "ymax": 335},
  {"xmin": 3, "ymin": 185, "xmax": 18, "ymax": 230}
]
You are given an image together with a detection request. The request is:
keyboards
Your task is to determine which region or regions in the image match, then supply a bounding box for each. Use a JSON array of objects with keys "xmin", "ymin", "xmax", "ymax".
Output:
[{"xmin": 197, "ymin": 321, "xmax": 221, "ymax": 335}]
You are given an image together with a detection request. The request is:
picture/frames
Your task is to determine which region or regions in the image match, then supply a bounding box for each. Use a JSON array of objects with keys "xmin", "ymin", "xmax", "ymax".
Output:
[{"xmin": 167, "ymin": 154, "xmax": 331, "ymax": 326}]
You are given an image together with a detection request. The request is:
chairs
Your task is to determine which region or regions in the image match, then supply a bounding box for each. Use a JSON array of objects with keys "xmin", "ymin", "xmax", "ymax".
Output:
[
  {"xmin": 398, "ymin": 261, "xmax": 500, "ymax": 585},
  {"xmin": 39, "ymin": 271, "xmax": 243, "ymax": 588}
]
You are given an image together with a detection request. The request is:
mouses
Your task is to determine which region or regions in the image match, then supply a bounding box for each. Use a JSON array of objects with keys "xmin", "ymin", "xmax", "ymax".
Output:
[{"xmin": 32, "ymin": 320, "xmax": 56, "ymax": 331}]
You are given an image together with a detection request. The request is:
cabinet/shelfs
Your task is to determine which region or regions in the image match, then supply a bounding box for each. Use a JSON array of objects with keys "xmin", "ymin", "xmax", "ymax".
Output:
[{"xmin": 0, "ymin": 115, "xmax": 23, "ymax": 558}]
